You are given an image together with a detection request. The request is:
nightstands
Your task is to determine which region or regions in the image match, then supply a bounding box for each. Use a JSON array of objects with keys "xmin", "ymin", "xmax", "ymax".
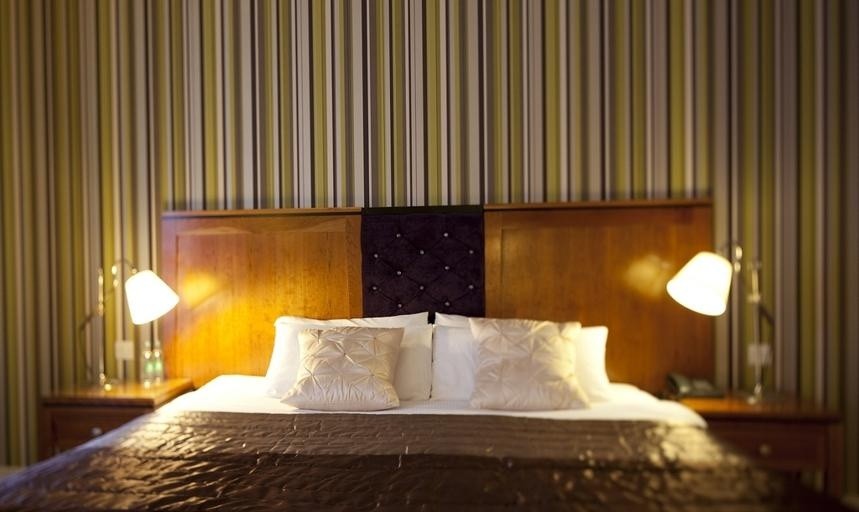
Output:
[
  {"xmin": 659, "ymin": 388, "xmax": 846, "ymax": 497},
  {"xmin": 35, "ymin": 383, "xmax": 192, "ymax": 463}
]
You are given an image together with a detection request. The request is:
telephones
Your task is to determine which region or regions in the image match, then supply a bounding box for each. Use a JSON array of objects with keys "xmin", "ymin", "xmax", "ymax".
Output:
[{"xmin": 668, "ymin": 374, "xmax": 722, "ymax": 397}]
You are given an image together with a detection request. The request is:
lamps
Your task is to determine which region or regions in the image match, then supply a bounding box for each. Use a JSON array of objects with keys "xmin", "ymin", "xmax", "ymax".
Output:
[
  {"xmin": 665, "ymin": 239, "xmax": 774, "ymax": 408},
  {"xmin": 68, "ymin": 254, "xmax": 181, "ymax": 393}
]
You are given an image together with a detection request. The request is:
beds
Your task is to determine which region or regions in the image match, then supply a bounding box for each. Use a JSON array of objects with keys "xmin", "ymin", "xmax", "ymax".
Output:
[{"xmin": 1, "ymin": 194, "xmax": 858, "ymax": 512}]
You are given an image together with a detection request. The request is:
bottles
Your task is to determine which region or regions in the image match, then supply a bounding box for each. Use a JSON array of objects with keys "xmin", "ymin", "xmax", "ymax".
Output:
[{"xmin": 142, "ymin": 341, "xmax": 162, "ymax": 388}]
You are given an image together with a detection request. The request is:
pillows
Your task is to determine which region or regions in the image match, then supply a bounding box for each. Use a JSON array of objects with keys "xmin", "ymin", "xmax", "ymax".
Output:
[
  {"xmin": 278, "ymin": 323, "xmax": 408, "ymax": 412},
  {"xmin": 262, "ymin": 310, "xmax": 429, "ymax": 399},
  {"xmin": 468, "ymin": 315, "xmax": 589, "ymax": 415},
  {"xmin": 430, "ymin": 323, "xmax": 610, "ymax": 407},
  {"xmin": 433, "ymin": 310, "xmax": 580, "ymax": 331}
]
